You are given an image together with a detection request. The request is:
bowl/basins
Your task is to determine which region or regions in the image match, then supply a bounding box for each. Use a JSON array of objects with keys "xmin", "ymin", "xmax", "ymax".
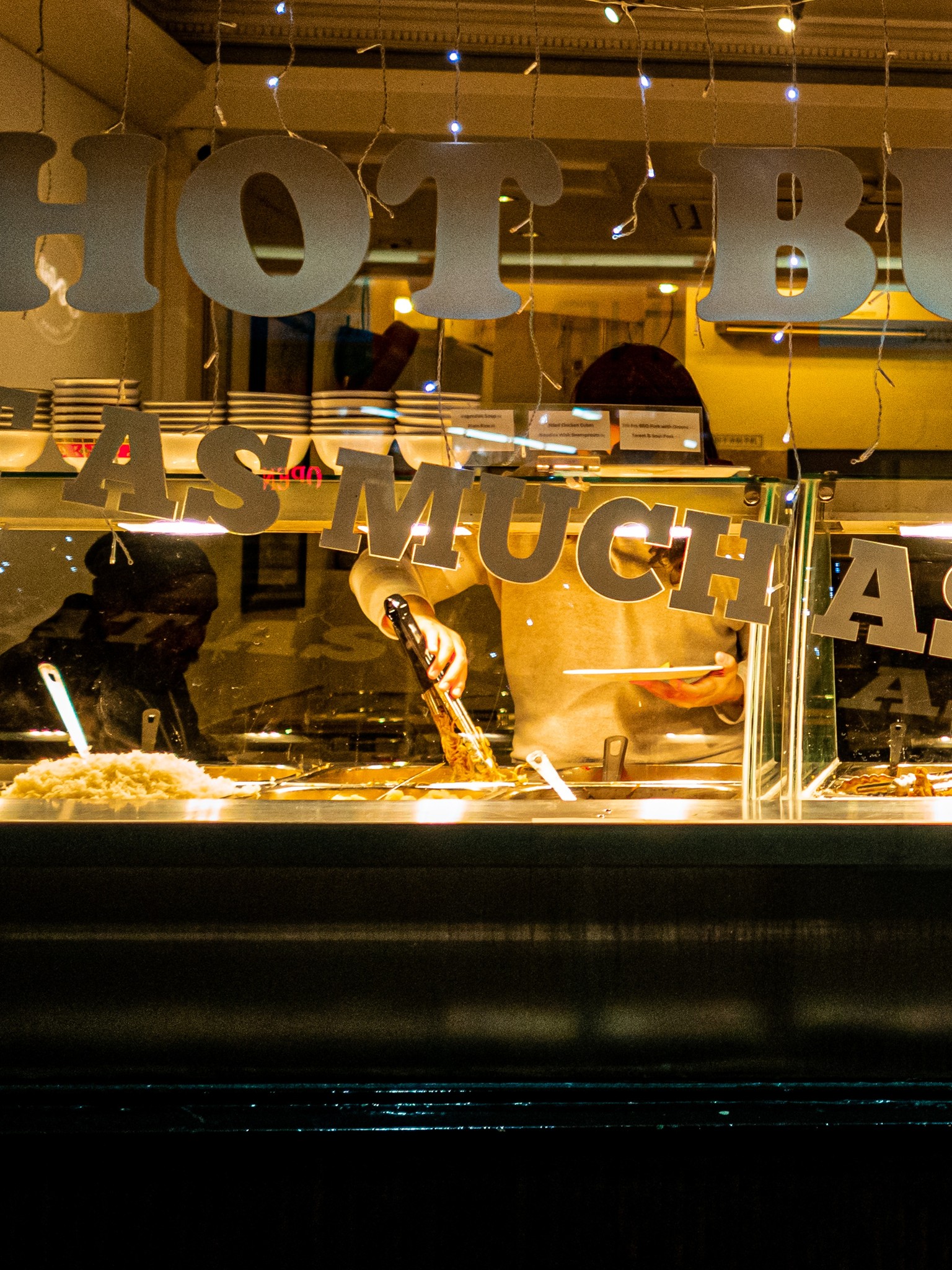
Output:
[{"xmin": 1, "ymin": 374, "xmax": 485, "ymax": 477}]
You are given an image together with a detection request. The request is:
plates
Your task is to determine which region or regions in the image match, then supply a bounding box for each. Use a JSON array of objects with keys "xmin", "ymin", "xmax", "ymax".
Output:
[{"xmin": 564, "ymin": 666, "xmax": 725, "ymax": 678}]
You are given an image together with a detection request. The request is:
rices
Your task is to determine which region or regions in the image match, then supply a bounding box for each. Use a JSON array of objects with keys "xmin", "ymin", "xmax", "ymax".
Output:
[{"xmin": 0, "ymin": 750, "xmax": 248, "ymax": 801}]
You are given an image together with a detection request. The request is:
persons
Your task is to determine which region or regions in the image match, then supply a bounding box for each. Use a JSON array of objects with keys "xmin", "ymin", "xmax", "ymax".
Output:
[
  {"xmin": 0, "ymin": 532, "xmax": 235, "ymax": 768},
  {"xmin": 350, "ymin": 344, "xmax": 825, "ymax": 767}
]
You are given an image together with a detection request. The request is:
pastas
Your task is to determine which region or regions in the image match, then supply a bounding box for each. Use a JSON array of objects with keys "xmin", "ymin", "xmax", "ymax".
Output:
[{"xmin": 441, "ymin": 726, "xmax": 525, "ymax": 782}]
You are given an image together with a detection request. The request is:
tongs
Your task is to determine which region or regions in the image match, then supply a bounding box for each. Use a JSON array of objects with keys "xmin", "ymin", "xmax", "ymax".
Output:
[{"xmin": 384, "ymin": 592, "xmax": 495, "ymax": 769}]
